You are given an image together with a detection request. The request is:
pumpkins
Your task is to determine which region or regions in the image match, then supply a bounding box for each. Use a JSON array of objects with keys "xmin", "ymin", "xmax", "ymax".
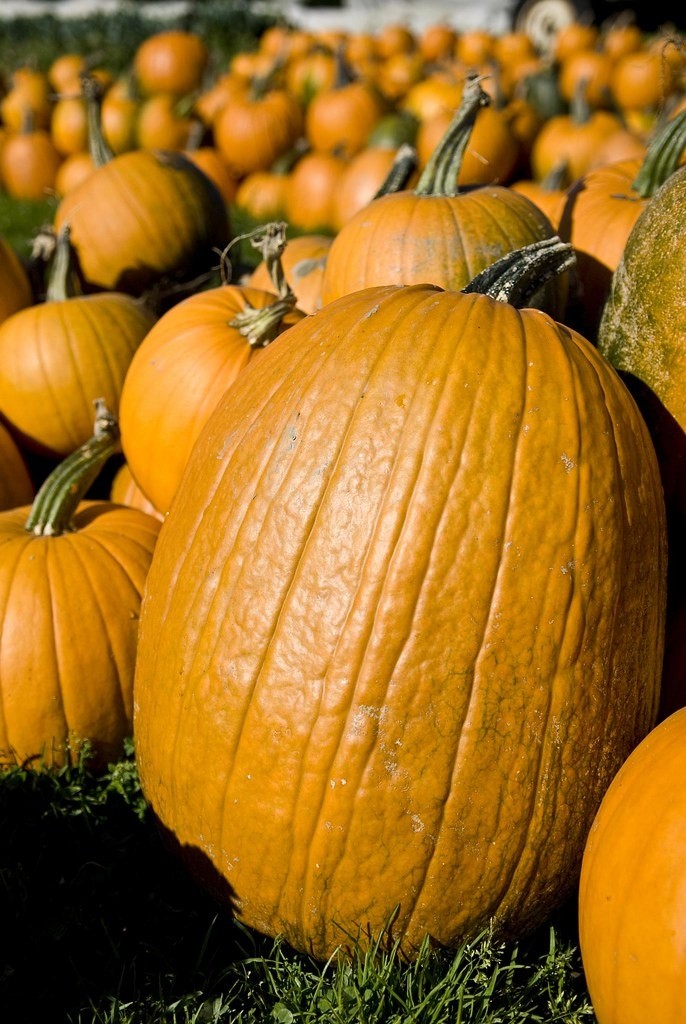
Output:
[{"xmin": 0, "ymin": 20, "xmax": 686, "ymax": 1024}]
